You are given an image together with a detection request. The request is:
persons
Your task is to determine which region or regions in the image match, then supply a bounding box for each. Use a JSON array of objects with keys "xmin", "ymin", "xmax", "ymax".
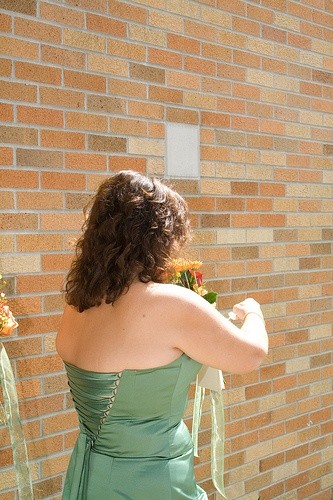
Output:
[{"xmin": 55, "ymin": 167, "xmax": 270, "ymax": 500}]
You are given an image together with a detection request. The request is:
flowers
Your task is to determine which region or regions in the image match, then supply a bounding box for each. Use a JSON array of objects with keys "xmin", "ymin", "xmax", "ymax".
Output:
[
  {"xmin": 167, "ymin": 258, "xmax": 219, "ymax": 307},
  {"xmin": 0, "ymin": 275, "xmax": 20, "ymax": 337}
]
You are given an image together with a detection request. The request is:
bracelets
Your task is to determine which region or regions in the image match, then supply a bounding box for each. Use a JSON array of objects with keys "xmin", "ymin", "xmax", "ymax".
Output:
[{"xmin": 244, "ymin": 313, "xmax": 263, "ymax": 323}]
